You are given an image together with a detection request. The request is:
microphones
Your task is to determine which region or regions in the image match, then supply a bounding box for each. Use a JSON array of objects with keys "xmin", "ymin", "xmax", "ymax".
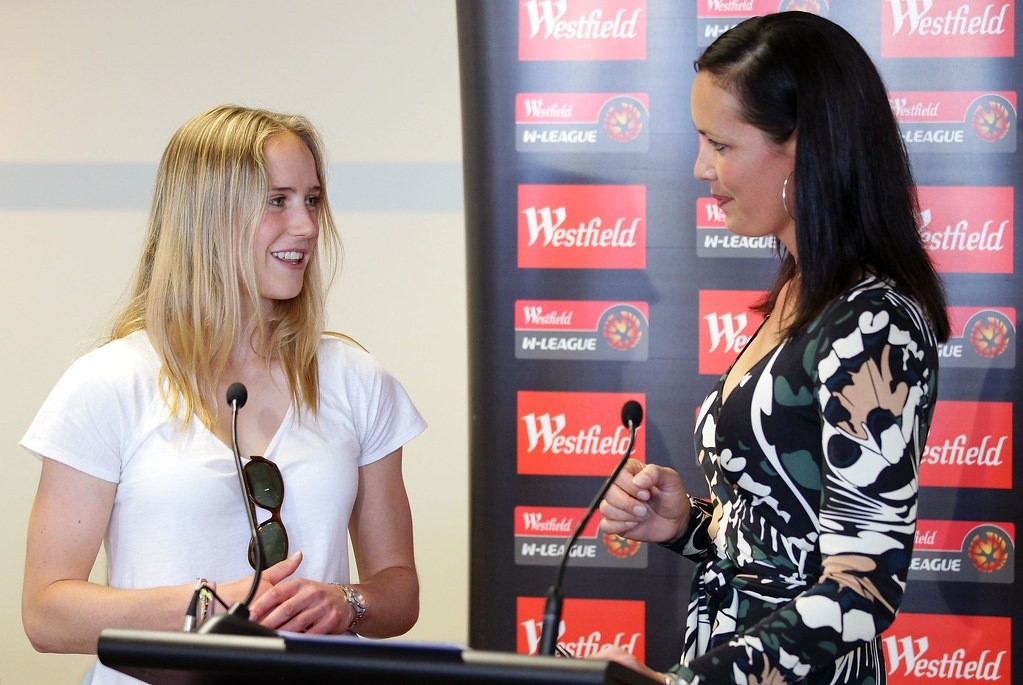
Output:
[
  {"xmin": 196, "ymin": 381, "xmax": 280, "ymax": 638},
  {"xmin": 537, "ymin": 401, "xmax": 644, "ymax": 656}
]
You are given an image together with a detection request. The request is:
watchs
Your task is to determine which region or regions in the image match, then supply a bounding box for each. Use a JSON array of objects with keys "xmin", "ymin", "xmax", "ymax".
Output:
[{"xmin": 330, "ymin": 582, "xmax": 367, "ymax": 632}]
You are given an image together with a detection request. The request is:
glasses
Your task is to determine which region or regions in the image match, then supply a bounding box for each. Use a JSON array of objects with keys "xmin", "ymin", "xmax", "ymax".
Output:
[{"xmin": 243, "ymin": 456, "xmax": 289, "ymax": 573}]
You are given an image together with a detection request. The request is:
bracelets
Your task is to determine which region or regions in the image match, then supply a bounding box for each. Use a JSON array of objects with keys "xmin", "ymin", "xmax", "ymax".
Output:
[{"xmin": 196, "ymin": 579, "xmax": 216, "ymax": 621}]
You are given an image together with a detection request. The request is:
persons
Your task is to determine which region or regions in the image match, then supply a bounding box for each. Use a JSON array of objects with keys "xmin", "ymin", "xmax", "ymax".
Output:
[
  {"xmin": 597, "ymin": 10, "xmax": 951, "ymax": 685},
  {"xmin": 20, "ymin": 106, "xmax": 427, "ymax": 685}
]
